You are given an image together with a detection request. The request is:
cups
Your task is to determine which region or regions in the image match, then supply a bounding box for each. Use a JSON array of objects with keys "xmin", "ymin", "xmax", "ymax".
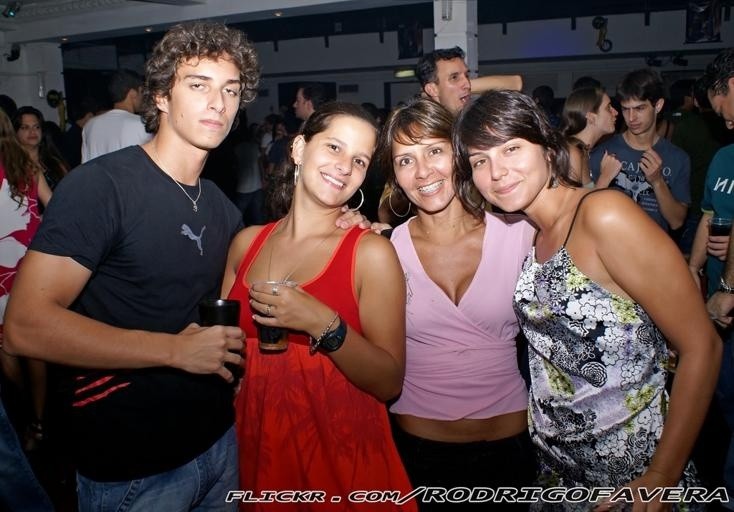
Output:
[
  {"xmin": 196, "ymin": 299, "xmax": 240, "ymax": 388},
  {"xmin": 706, "ymin": 217, "xmax": 733, "ymax": 236},
  {"xmin": 247, "ymin": 280, "xmax": 297, "ymax": 352}
]
